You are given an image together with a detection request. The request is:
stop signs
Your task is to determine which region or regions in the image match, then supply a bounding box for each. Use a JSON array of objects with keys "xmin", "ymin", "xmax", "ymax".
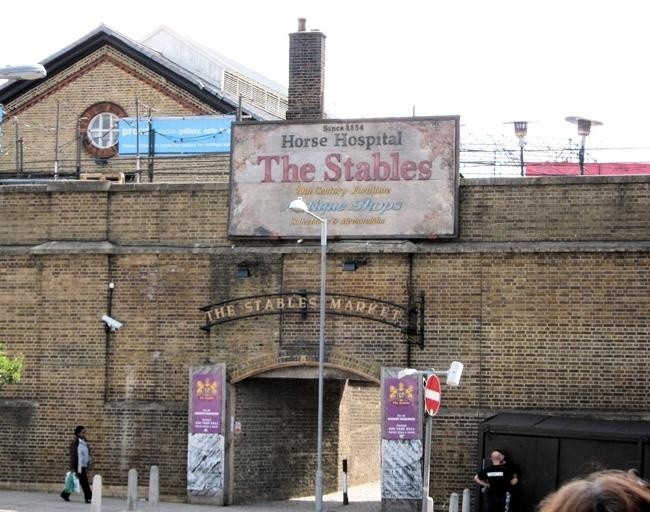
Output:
[{"xmin": 425, "ymin": 375, "xmax": 442, "ymax": 416}]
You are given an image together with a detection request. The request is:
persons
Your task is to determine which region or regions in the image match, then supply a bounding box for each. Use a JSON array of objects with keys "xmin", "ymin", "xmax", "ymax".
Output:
[
  {"xmin": 59, "ymin": 425, "xmax": 92, "ymax": 503},
  {"xmin": 536, "ymin": 467, "xmax": 650, "ymax": 511},
  {"xmin": 626, "ymin": 468, "xmax": 650, "ymax": 489},
  {"xmin": 495, "ymin": 448, "xmax": 518, "ymax": 511},
  {"xmin": 473, "ymin": 450, "xmax": 517, "ymax": 512}
]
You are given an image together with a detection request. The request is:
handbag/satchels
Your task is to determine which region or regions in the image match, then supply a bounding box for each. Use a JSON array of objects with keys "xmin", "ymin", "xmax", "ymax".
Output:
[{"xmin": 65, "ymin": 471, "xmax": 82, "ymax": 494}]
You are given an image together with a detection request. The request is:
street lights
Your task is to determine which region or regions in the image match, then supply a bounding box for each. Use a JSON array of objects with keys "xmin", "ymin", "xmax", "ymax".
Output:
[{"xmin": 288, "ymin": 197, "xmax": 326, "ymax": 511}]
